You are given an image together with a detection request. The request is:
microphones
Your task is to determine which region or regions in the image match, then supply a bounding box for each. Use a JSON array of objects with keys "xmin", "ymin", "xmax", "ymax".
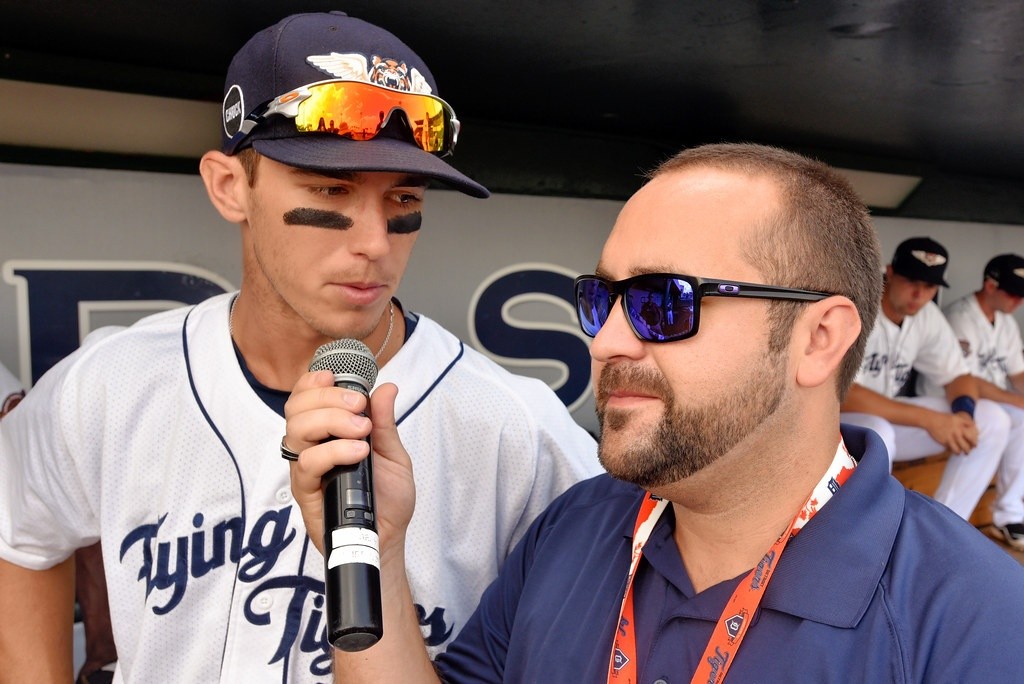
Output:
[{"xmin": 308, "ymin": 339, "xmax": 384, "ymax": 652}]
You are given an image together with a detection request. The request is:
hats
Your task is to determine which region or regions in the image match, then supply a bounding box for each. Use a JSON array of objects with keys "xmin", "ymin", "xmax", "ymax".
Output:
[
  {"xmin": 222, "ymin": 12, "xmax": 491, "ymax": 200},
  {"xmin": 985, "ymin": 254, "xmax": 1024, "ymax": 296},
  {"xmin": 893, "ymin": 238, "xmax": 949, "ymax": 287}
]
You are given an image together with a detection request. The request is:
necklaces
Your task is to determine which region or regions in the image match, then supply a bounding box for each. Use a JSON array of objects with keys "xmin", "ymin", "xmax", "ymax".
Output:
[{"xmin": 229, "ymin": 292, "xmax": 394, "ymax": 360}]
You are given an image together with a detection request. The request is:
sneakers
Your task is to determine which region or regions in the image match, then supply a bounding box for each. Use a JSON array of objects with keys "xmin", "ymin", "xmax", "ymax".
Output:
[{"xmin": 991, "ymin": 520, "xmax": 1024, "ymax": 549}]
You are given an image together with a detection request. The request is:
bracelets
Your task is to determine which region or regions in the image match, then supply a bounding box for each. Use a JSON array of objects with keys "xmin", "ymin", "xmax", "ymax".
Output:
[{"xmin": 952, "ymin": 395, "xmax": 976, "ymax": 419}]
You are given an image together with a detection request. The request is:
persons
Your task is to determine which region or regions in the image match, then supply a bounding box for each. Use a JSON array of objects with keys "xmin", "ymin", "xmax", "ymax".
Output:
[
  {"xmin": 579, "ymin": 290, "xmax": 607, "ymax": 335},
  {"xmin": 839, "ymin": 235, "xmax": 1012, "ymax": 521},
  {"xmin": 941, "ymin": 253, "xmax": 1023, "ymax": 549},
  {"xmin": 280, "ymin": 142, "xmax": 1024, "ymax": 684},
  {"xmin": 642, "ymin": 291, "xmax": 674, "ymax": 335},
  {"xmin": 0, "ymin": 9, "xmax": 610, "ymax": 684},
  {"xmin": 0, "ymin": 364, "xmax": 118, "ymax": 684}
]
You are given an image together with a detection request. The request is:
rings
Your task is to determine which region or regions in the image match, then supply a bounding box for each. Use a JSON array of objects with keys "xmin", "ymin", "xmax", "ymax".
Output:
[{"xmin": 280, "ymin": 435, "xmax": 299, "ymax": 461}]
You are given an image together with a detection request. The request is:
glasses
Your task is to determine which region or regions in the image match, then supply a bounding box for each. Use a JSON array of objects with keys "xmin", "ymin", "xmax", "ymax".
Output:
[
  {"xmin": 573, "ymin": 273, "xmax": 830, "ymax": 343},
  {"xmin": 224, "ymin": 79, "xmax": 461, "ymax": 157}
]
